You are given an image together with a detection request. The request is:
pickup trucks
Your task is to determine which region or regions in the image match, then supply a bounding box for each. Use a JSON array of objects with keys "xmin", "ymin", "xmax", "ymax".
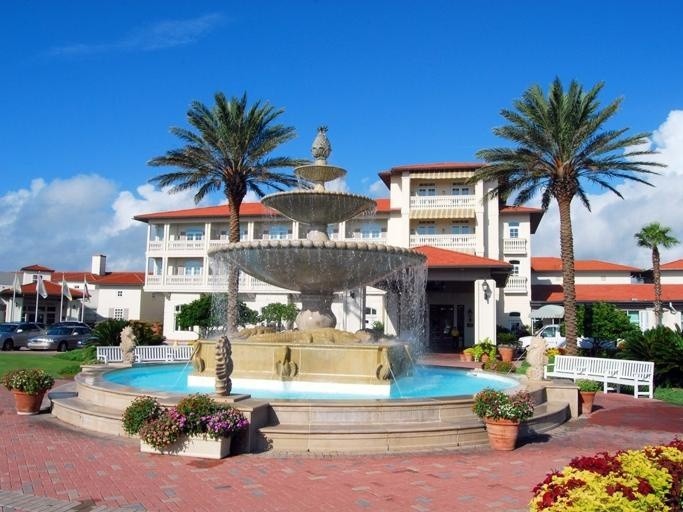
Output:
[{"xmin": 519, "ymin": 324, "xmax": 616, "ymax": 349}]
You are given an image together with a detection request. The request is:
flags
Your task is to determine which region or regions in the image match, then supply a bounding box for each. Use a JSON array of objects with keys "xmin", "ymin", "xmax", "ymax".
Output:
[
  {"xmin": 11, "ymin": 274, "xmax": 21, "ymax": 295},
  {"xmin": 85, "ymin": 280, "xmax": 92, "ymax": 301},
  {"xmin": 35, "ymin": 275, "xmax": 48, "ymax": 299},
  {"xmin": 60, "ymin": 278, "xmax": 72, "ymax": 302}
]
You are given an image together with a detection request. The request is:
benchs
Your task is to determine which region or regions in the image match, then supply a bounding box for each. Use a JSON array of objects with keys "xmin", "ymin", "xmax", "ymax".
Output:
[{"xmin": 542, "ymin": 354, "xmax": 654, "ymax": 399}]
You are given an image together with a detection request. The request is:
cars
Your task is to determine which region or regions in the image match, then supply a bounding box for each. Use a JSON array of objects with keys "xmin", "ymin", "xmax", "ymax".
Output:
[{"xmin": 1, "ymin": 321, "xmax": 100, "ymax": 352}]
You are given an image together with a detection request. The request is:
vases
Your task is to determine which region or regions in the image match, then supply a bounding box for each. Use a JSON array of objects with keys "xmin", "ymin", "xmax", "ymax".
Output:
[
  {"xmin": 138, "ymin": 430, "xmax": 233, "ymax": 459},
  {"xmin": 483, "ymin": 416, "xmax": 523, "ymax": 451}
]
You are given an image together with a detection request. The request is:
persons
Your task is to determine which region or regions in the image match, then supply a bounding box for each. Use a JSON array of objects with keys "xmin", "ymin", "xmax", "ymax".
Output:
[{"xmin": 451, "ymin": 326, "xmax": 460, "ymax": 349}]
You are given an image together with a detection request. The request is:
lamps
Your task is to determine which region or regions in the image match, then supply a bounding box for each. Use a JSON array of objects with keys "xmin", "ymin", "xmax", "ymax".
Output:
[{"xmin": 480, "ymin": 279, "xmax": 490, "ymax": 301}]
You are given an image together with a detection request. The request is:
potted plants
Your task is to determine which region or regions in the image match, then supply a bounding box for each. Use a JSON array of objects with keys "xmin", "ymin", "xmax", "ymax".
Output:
[
  {"xmin": 575, "ymin": 378, "xmax": 601, "ymax": 415},
  {"xmin": 462, "ymin": 333, "xmax": 518, "ymax": 363},
  {"xmin": 2, "ymin": 367, "xmax": 54, "ymax": 416}
]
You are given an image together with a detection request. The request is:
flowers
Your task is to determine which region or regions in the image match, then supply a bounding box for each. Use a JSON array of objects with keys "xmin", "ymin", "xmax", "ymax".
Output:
[
  {"xmin": 472, "ymin": 388, "xmax": 534, "ymax": 423},
  {"xmin": 117, "ymin": 392, "xmax": 250, "ymax": 452}
]
